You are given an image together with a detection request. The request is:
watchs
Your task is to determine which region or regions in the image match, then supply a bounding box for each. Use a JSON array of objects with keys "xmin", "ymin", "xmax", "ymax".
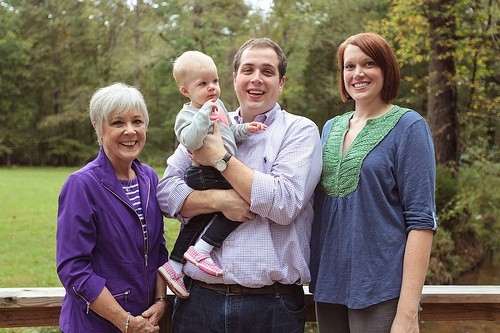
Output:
[{"xmin": 215, "ymin": 151, "xmax": 232, "ymax": 172}]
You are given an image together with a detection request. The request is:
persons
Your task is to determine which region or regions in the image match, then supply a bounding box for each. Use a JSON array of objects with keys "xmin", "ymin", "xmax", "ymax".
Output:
[
  {"xmin": 309, "ymin": 32, "xmax": 438, "ymax": 333},
  {"xmin": 157, "ymin": 38, "xmax": 323, "ymax": 333},
  {"xmin": 157, "ymin": 51, "xmax": 268, "ymax": 298},
  {"xmin": 56, "ymin": 81, "xmax": 169, "ymax": 333}
]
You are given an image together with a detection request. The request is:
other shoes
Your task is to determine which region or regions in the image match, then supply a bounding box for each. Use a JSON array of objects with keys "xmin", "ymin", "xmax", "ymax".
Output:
[
  {"xmin": 183, "ymin": 245, "xmax": 223, "ymax": 277},
  {"xmin": 158, "ymin": 262, "xmax": 190, "ymax": 299}
]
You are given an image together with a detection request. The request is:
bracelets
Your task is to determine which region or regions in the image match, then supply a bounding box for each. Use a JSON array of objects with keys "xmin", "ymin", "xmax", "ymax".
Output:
[
  {"xmin": 126, "ymin": 312, "xmax": 131, "ymax": 332},
  {"xmin": 155, "ymin": 296, "xmax": 168, "ymax": 301}
]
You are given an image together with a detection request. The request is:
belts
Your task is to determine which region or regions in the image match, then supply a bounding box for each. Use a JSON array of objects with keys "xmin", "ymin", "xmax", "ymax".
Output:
[{"xmin": 183, "ymin": 274, "xmax": 303, "ymax": 295}]
